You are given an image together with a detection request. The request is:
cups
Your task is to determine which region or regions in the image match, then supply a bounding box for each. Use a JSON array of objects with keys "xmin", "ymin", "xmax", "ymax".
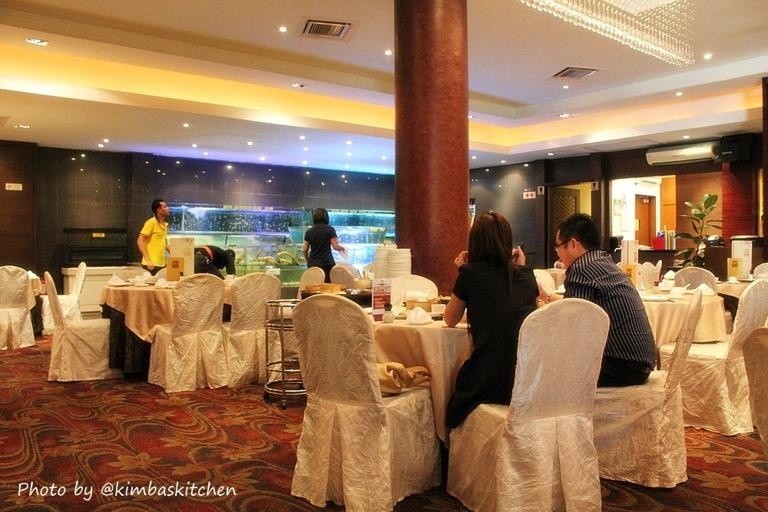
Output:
[
  {"xmin": 673, "ymin": 287, "xmax": 686, "ymax": 295},
  {"xmin": 729, "ymin": 277, "xmax": 737, "ymax": 282},
  {"xmin": 431, "ymin": 303, "xmax": 447, "ymax": 313},
  {"xmin": 715, "ymin": 277, "xmax": 719, "ymax": 281}
]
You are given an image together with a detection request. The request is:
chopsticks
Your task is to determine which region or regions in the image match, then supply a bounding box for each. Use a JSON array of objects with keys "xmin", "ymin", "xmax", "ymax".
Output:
[{"xmin": 513, "ymin": 241, "xmax": 537, "ymax": 263}]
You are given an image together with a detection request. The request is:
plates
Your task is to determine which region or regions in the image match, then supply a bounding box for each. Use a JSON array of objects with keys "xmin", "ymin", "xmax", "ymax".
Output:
[
  {"xmin": 109, "ymin": 278, "xmax": 176, "ymax": 289},
  {"xmin": 410, "ymin": 320, "xmax": 432, "ymax": 325},
  {"xmin": 716, "ymin": 281, "xmax": 722, "ymax": 284},
  {"xmin": 554, "ymin": 290, "xmax": 566, "ymax": 295},
  {"xmin": 644, "ymin": 294, "xmax": 670, "ymax": 301},
  {"xmin": 728, "ymin": 281, "xmax": 740, "ymax": 284},
  {"xmin": 375, "ymin": 247, "xmax": 411, "ymax": 280}
]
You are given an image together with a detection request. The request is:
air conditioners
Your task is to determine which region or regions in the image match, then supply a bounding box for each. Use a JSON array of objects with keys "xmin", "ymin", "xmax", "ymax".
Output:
[{"xmin": 645, "ymin": 142, "xmax": 722, "ymax": 167}]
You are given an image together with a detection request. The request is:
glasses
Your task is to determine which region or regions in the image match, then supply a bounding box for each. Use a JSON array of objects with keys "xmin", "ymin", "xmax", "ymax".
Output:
[{"xmin": 553, "ymin": 239, "xmax": 576, "ymax": 249}]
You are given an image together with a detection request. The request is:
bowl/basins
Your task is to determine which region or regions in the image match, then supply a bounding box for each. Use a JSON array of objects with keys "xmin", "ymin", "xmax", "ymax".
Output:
[
  {"xmin": 440, "ymin": 299, "xmax": 449, "ymax": 304},
  {"xmin": 406, "ymin": 300, "xmax": 438, "ymax": 312}
]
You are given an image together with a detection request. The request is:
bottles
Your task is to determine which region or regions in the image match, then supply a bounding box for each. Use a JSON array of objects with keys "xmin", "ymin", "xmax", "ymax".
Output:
[
  {"xmin": 382, "ymin": 303, "xmax": 395, "ymax": 322},
  {"xmin": 356, "ymin": 278, "xmax": 372, "ymax": 288},
  {"xmin": 667, "ymin": 230, "xmax": 676, "ymax": 250}
]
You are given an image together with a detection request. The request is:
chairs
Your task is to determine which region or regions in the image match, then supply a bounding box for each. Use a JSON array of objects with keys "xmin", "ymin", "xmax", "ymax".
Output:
[
  {"xmin": 0, "ymin": 261, "xmax": 283, "ymax": 395},
  {"xmin": 288, "ymin": 260, "xmax": 768, "ymax": 512}
]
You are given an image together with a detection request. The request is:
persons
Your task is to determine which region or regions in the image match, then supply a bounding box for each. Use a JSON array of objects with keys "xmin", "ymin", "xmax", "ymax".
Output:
[
  {"xmin": 194, "ymin": 244, "xmax": 236, "ymax": 280},
  {"xmin": 137, "ymin": 199, "xmax": 171, "ymax": 276},
  {"xmin": 537, "ymin": 213, "xmax": 656, "ymax": 388},
  {"xmin": 445, "ymin": 210, "xmax": 539, "ymax": 429},
  {"xmin": 303, "ymin": 207, "xmax": 345, "ymax": 283}
]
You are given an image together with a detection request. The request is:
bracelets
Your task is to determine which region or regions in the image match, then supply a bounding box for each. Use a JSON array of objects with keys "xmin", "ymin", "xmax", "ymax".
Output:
[{"xmin": 544, "ymin": 294, "xmax": 550, "ymax": 303}]
